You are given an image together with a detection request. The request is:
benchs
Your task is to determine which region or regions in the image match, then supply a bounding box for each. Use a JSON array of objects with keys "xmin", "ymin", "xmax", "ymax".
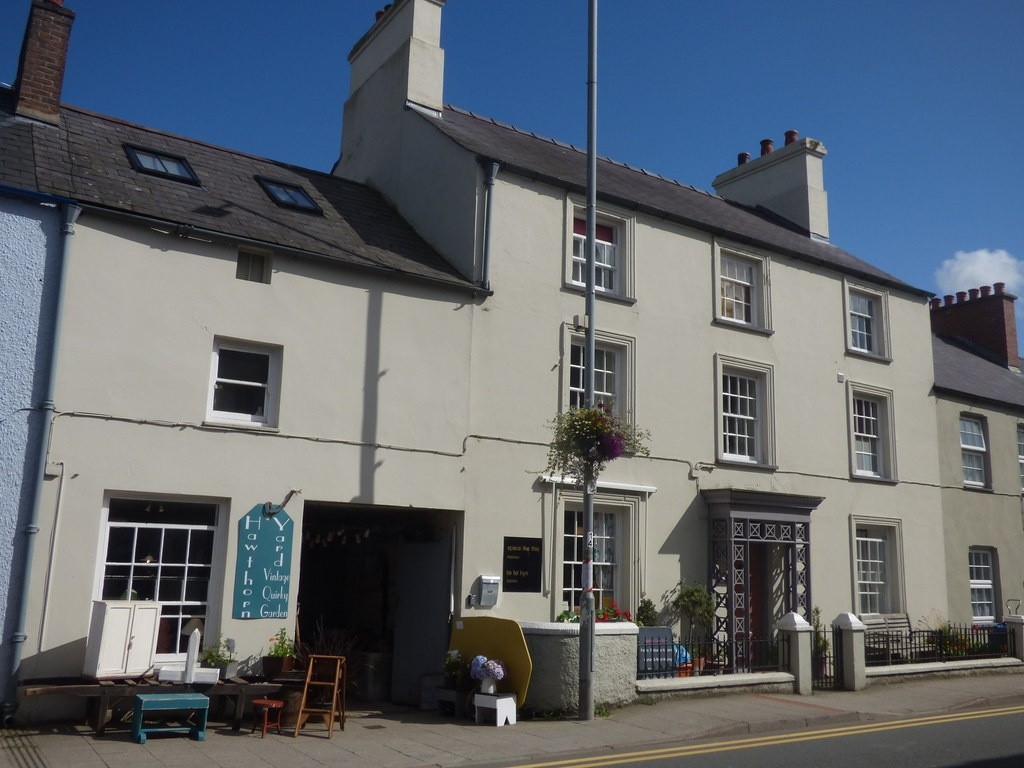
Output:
[{"xmin": 860, "ymin": 613, "xmax": 941, "ymax": 664}]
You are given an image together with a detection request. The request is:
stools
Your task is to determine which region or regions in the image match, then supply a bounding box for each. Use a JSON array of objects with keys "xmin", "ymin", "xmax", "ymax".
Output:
[
  {"xmin": 132, "ymin": 692, "xmax": 210, "ymax": 744},
  {"xmin": 473, "ymin": 694, "xmax": 518, "ymax": 726},
  {"xmin": 437, "ymin": 687, "xmax": 469, "ymax": 721},
  {"xmin": 250, "ymin": 701, "xmax": 284, "ymax": 738}
]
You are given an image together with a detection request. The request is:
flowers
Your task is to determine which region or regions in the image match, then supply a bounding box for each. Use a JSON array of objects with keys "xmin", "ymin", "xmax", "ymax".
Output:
[
  {"xmin": 928, "ymin": 621, "xmax": 1007, "ymax": 657},
  {"xmin": 529, "ymin": 399, "xmax": 651, "ymax": 485},
  {"xmin": 261, "ymin": 627, "xmax": 293, "ymax": 655},
  {"xmin": 468, "ymin": 654, "xmax": 507, "ymax": 680},
  {"xmin": 556, "ymin": 597, "xmax": 631, "ymax": 623},
  {"xmin": 440, "ymin": 650, "xmax": 467, "ymax": 675}
]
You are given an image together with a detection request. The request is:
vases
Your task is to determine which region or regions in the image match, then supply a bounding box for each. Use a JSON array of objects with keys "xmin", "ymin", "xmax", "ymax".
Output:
[
  {"xmin": 480, "ymin": 676, "xmax": 497, "ymax": 695},
  {"xmin": 444, "ymin": 675, "xmax": 458, "ymax": 690},
  {"xmin": 261, "ymin": 656, "xmax": 293, "ymax": 679},
  {"xmin": 677, "ymin": 664, "xmax": 693, "ymax": 678}
]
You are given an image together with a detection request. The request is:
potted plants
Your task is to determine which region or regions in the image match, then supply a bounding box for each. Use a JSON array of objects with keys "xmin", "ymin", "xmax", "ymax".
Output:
[
  {"xmin": 810, "ymin": 607, "xmax": 829, "ymax": 677},
  {"xmin": 676, "ymin": 581, "xmax": 714, "ymax": 675},
  {"xmin": 202, "ymin": 635, "xmax": 239, "ymax": 680}
]
